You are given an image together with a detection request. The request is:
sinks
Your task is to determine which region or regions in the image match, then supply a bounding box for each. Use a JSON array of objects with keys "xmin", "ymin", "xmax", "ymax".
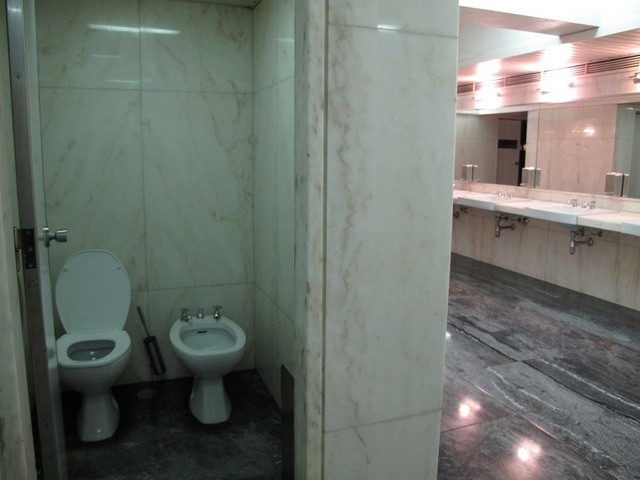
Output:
[
  {"xmin": 175, "ymin": 314, "xmax": 242, "ymax": 354},
  {"xmin": 474, "ymin": 195, "xmax": 516, "ymax": 202},
  {"xmin": 541, "ymin": 204, "xmax": 597, "ymax": 214}
]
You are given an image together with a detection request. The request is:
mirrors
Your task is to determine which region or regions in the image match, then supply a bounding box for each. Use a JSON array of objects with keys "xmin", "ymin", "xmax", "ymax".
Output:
[{"xmin": 454, "ymin": 99, "xmax": 640, "ymax": 198}]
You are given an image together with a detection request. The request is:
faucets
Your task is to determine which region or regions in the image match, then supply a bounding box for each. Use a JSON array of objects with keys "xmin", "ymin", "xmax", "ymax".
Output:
[
  {"xmin": 583, "ymin": 200, "xmax": 596, "ymax": 209},
  {"xmin": 494, "ymin": 189, "xmax": 502, "ymax": 197},
  {"xmin": 567, "ymin": 197, "xmax": 579, "ymax": 207},
  {"xmin": 213, "ymin": 306, "xmax": 223, "ymax": 318},
  {"xmin": 181, "ymin": 308, "xmax": 192, "ymax": 320}
]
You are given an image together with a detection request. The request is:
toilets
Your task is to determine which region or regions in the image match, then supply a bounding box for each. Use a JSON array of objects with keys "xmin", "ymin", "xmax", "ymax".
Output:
[{"xmin": 54, "ymin": 250, "xmax": 132, "ymax": 442}]
